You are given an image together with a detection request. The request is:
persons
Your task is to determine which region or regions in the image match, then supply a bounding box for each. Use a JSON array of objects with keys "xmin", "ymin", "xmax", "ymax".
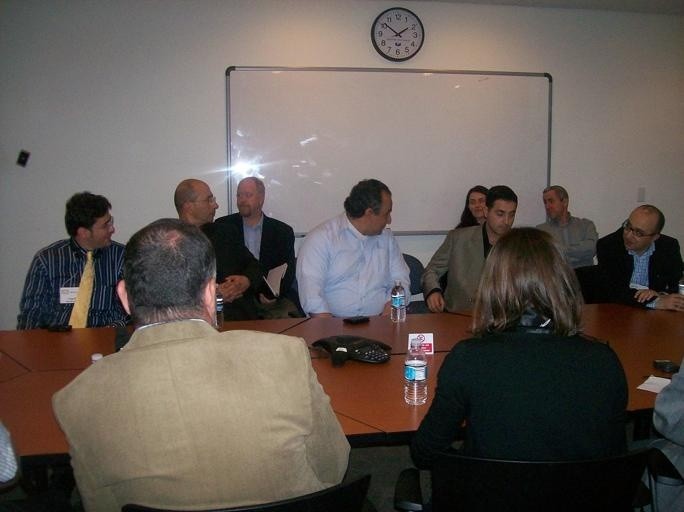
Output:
[
  {"xmin": 215, "ymin": 178, "xmax": 306, "ymax": 316},
  {"xmin": 175, "ymin": 178, "xmax": 261, "ymax": 318},
  {"xmin": 17, "ymin": 191, "xmax": 134, "ymax": 329},
  {"xmin": 421, "ymin": 182, "xmax": 683, "ymax": 317},
  {"xmin": 51, "ymin": 218, "xmax": 352, "ymax": 512},
  {"xmin": 295, "ymin": 179, "xmax": 410, "ymax": 318},
  {"xmin": 409, "ymin": 227, "xmax": 629, "ymax": 470},
  {"xmin": 632, "ymin": 355, "xmax": 684, "ymax": 512}
]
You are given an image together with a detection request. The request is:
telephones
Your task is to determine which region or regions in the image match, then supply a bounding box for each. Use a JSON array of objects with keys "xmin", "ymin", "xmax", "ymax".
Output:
[{"xmin": 311, "ymin": 335, "xmax": 392, "ymax": 366}]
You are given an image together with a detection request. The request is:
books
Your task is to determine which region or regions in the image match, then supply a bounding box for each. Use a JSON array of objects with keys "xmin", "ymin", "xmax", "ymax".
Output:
[{"xmin": 262, "ymin": 263, "xmax": 287, "ymax": 300}]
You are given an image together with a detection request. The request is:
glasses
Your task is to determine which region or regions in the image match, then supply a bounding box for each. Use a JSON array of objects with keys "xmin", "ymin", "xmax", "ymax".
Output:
[
  {"xmin": 196, "ymin": 196, "xmax": 216, "ymax": 205},
  {"xmin": 621, "ymin": 219, "xmax": 656, "ymax": 238},
  {"xmin": 97, "ymin": 216, "xmax": 114, "ymax": 229}
]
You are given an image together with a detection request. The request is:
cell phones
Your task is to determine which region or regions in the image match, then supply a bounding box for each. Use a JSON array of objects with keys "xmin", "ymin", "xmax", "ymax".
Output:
[
  {"xmin": 47, "ymin": 325, "xmax": 72, "ymax": 333},
  {"xmin": 342, "ymin": 316, "xmax": 369, "ymax": 325},
  {"xmin": 653, "ymin": 359, "xmax": 680, "ymax": 373}
]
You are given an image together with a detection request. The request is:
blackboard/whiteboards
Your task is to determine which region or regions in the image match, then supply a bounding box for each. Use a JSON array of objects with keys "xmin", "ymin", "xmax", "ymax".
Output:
[{"xmin": 225, "ymin": 66, "xmax": 553, "ymax": 238}]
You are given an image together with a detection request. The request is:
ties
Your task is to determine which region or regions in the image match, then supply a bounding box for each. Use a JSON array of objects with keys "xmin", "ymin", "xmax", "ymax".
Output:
[{"xmin": 68, "ymin": 251, "xmax": 95, "ymax": 330}]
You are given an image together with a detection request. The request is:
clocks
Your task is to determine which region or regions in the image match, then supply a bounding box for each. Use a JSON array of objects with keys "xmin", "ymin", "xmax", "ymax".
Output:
[{"xmin": 371, "ymin": 7, "xmax": 425, "ymax": 61}]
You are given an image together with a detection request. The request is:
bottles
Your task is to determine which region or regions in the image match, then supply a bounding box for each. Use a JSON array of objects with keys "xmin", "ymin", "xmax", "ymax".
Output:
[
  {"xmin": 678, "ymin": 271, "xmax": 684, "ymax": 294},
  {"xmin": 216, "ymin": 283, "xmax": 225, "ymax": 328},
  {"xmin": 91, "ymin": 352, "xmax": 102, "ymax": 362},
  {"xmin": 402, "ymin": 340, "xmax": 430, "ymax": 407},
  {"xmin": 389, "ymin": 282, "xmax": 407, "ymax": 324}
]
